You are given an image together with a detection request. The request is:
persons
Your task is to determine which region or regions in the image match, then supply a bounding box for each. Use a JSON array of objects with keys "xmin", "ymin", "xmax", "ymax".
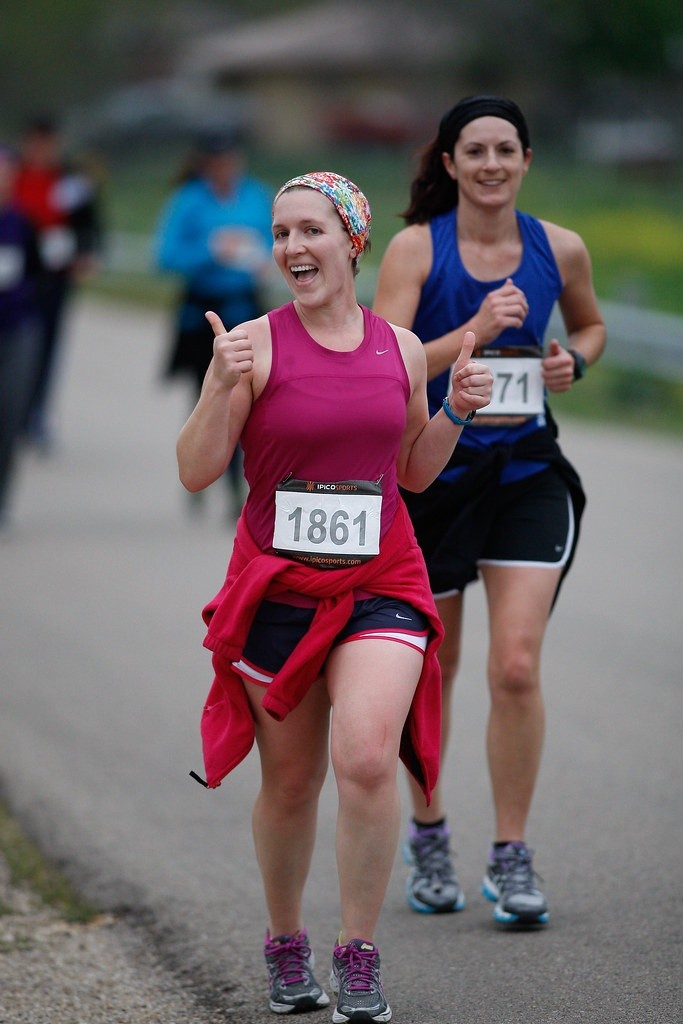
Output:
[
  {"xmin": 174, "ymin": 170, "xmax": 495, "ymax": 1024},
  {"xmin": 1, "ymin": 141, "xmax": 48, "ymax": 506},
  {"xmin": 4, "ymin": 110, "xmax": 102, "ymax": 455},
  {"xmin": 369, "ymin": 94, "xmax": 608, "ymax": 927},
  {"xmin": 152, "ymin": 128, "xmax": 276, "ymax": 533}
]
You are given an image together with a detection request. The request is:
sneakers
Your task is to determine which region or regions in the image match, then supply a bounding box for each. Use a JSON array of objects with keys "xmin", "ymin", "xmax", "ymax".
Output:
[
  {"xmin": 404, "ymin": 814, "xmax": 549, "ymax": 930},
  {"xmin": 262, "ymin": 930, "xmax": 393, "ymax": 1024}
]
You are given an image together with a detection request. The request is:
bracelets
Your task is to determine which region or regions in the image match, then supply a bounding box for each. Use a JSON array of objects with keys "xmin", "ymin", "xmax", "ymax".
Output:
[
  {"xmin": 565, "ymin": 348, "xmax": 587, "ymax": 384},
  {"xmin": 443, "ymin": 397, "xmax": 476, "ymax": 426}
]
